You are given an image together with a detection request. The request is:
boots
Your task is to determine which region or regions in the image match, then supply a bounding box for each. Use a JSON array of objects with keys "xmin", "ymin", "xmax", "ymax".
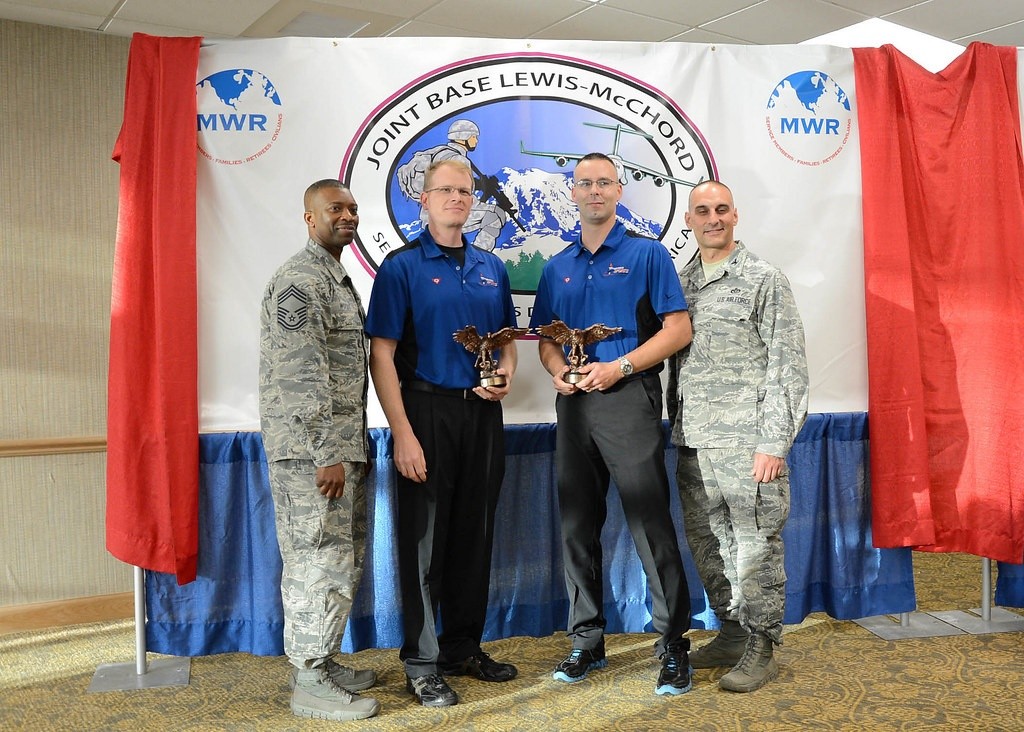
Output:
[
  {"xmin": 687, "ymin": 619, "xmax": 751, "ymax": 669},
  {"xmin": 719, "ymin": 631, "xmax": 780, "ymax": 693}
]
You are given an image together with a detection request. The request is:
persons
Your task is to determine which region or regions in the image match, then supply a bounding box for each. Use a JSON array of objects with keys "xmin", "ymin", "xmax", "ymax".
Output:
[
  {"xmin": 258, "ymin": 179, "xmax": 380, "ymax": 721},
  {"xmin": 528, "ymin": 152, "xmax": 693, "ymax": 696},
  {"xmin": 663, "ymin": 180, "xmax": 809, "ymax": 693},
  {"xmin": 363, "ymin": 162, "xmax": 517, "ymax": 706}
]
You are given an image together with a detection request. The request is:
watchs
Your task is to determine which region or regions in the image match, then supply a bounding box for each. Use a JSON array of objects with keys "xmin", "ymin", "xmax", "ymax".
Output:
[{"xmin": 618, "ymin": 357, "xmax": 632, "ymax": 377}]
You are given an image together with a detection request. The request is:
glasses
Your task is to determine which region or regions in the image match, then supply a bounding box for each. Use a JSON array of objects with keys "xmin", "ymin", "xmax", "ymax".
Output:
[
  {"xmin": 424, "ymin": 185, "xmax": 473, "ymax": 196},
  {"xmin": 574, "ymin": 180, "xmax": 619, "ymax": 189}
]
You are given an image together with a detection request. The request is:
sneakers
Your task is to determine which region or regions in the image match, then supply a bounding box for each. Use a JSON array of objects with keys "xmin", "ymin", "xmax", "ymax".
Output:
[
  {"xmin": 441, "ymin": 647, "xmax": 517, "ymax": 682},
  {"xmin": 288, "ymin": 656, "xmax": 377, "ymax": 692},
  {"xmin": 553, "ymin": 641, "xmax": 607, "ymax": 682},
  {"xmin": 289, "ymin": 668, "xmax": 381, "ymax": 721},
  {"xmin": 655, "ymin": 643, "xmax": 694, "ymax": 694},
  {"xmin": 405, "ymin": 672, "xmax": 458, "ymax": 707}
]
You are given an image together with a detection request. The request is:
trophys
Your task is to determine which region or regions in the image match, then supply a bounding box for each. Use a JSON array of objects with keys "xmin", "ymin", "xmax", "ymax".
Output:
[
  {"xmin": 452, "ymin": 325, "xmax": 532, "ymax": 387},
  {"xmin": 535, "ymin": 320, "xmax": 623, "ymax": 384}
]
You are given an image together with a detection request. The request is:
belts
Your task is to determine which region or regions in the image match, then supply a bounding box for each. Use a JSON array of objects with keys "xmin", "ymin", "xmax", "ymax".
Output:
[{"xmin": 400, "ymin": 380, "xmax": 483, "ymax": 401}]
